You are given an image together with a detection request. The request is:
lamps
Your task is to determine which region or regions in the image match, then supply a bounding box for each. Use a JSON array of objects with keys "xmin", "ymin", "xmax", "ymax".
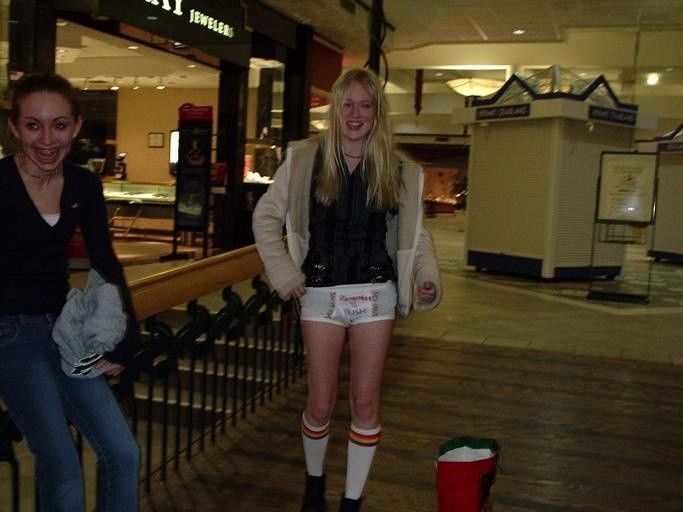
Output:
[{"xmin": 445, "ymin": 76, "xmax": 505, "ymax": 98}]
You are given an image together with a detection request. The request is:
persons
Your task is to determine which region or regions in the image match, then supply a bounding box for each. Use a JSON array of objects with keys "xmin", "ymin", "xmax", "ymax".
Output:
[
  {"xmin": 249, "ymin": 61, "xmax": 443, "ymax": 511},
  {"xmin": 0, "ymin": 66, "xmax": 148, "ymax": 510}
]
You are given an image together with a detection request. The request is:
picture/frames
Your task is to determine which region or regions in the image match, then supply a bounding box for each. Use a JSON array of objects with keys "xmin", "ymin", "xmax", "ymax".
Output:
[{"xmin": 147, "ymin": 131, "xmax": 164, "ymax": 148}]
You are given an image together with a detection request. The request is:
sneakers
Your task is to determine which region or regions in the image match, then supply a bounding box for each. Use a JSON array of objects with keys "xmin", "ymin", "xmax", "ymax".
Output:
[
  {"xmin": 302, "ymin": 471, "xmax": 326, "ymax": 512},
  {"xmin": 339, "ymin": 492, "xmax": 363, "ymax": 512}
]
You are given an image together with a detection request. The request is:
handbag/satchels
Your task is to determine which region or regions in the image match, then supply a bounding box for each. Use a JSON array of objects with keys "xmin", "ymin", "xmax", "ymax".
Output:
[{"xmin": 433, "ymin": 436, "xmax": 504, "ymax": 512}]
